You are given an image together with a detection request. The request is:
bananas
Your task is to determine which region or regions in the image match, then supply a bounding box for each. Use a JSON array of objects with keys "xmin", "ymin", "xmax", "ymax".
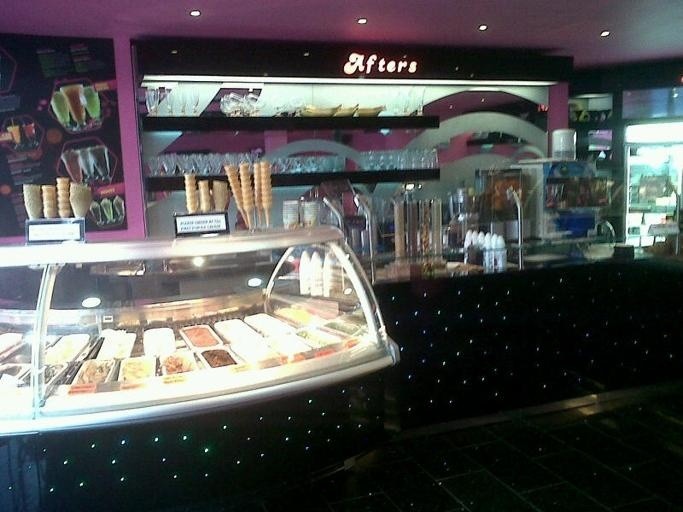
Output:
[{"xmin": 300, "ymin": 104, "xmax": 386, "ymax": 117}]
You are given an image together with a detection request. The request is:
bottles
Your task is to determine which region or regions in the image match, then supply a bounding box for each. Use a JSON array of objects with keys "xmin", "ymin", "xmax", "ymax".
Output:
[{"xmin": 463, "ymin": 228, "xmax": 509, "ymax": 271}]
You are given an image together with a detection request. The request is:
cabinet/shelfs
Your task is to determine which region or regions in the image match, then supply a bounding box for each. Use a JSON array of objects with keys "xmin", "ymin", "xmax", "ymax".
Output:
[
  {"xmin": 0, "ymin": 226, "xmax": 402, "ymax": 440},
  {"xmin": 143, "ymin": 113, "xmax": 440, "ymax": 191}
]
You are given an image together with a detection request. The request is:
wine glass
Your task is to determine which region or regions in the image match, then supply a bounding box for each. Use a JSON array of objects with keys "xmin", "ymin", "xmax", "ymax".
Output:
[
  {"xmin": 50, "ymin": 82, "xmax": 103, "ymax": 131},
  {"xmin": 59, "ymin": 144, "xmax": 112, "ymax": 185},
  {"xmin": 90, "ymin": 201, "xmax": 125, "ymax": 226}
]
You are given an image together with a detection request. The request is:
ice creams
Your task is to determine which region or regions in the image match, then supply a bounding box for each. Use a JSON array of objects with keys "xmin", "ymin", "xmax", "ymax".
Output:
[
  {"xmin": 89, "ymin": 195, "xmax": 125, "ymax": 221},
  {"xmin": 61, "ymin": 145, "xmax": 110, "ymax": 183}
]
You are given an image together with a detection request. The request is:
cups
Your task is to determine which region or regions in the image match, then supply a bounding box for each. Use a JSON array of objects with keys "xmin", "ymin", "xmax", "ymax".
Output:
[
  {"xmin": 145, "ymin": 89, "xmax": 201, "ymax": 117},
  {"xmin": 146, "ymin": 150, "xmax": 261, "ymax": 177},
  {"xmin": 7, "ymin": 121, "xmax": 39, "ymax": 152}
]
